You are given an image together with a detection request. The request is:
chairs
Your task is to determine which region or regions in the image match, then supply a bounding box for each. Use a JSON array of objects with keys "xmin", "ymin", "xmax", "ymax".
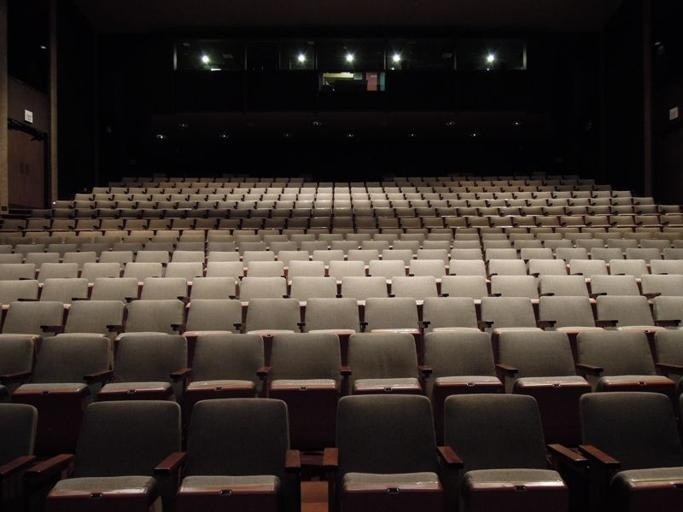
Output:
[{"xmin": 0, "ymin": 174, "xmax": 682, "ymax": 510}]
